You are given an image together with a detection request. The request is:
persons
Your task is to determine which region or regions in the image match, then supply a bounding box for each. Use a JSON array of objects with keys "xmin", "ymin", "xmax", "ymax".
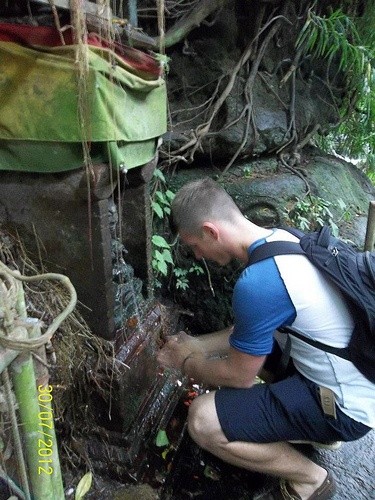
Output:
[{"xmin": 156, "ymin": 176, "xmax": 375, "ymax": 500}]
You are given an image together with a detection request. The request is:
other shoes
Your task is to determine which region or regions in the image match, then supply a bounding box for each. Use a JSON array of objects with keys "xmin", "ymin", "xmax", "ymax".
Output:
[
  {"xmin": 289, "ymin": 437, "xmax": 340, "ymax": 451},
  {"xmin": 252, "ymin": 466, "xmax": 339, "ymax": 500}
]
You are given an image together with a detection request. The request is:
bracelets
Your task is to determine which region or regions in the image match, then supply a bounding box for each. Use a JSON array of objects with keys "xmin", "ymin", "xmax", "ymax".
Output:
[{"xmin": 181, "ymin": 355, "xmax": 195, "ymax": 377}]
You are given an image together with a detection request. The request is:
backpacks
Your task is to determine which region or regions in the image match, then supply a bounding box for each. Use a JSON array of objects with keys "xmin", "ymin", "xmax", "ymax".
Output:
[{"xmin": 246, "ymin": 223, "xmax": 374, "ymax": 383}]
